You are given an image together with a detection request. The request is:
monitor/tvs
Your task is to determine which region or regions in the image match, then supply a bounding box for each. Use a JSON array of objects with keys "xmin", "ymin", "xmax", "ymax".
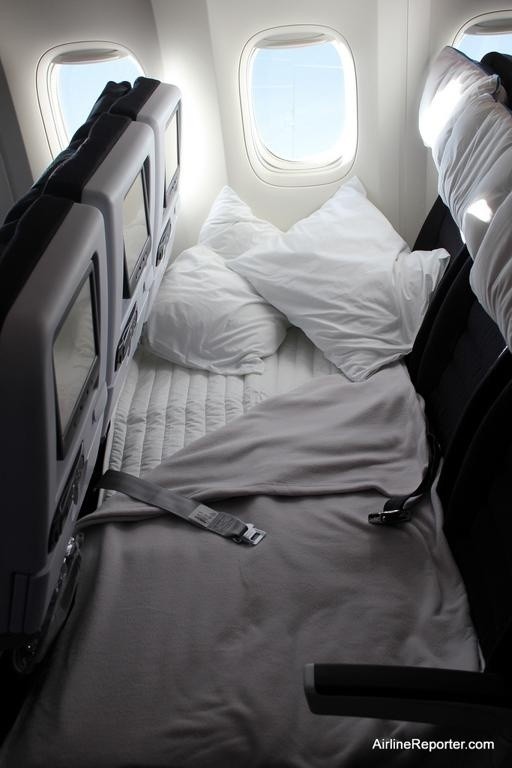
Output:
[
  {"xmin": 52, "ymin": 259, "xmax": 100, "ymax": 461},
  {"xmin": 163, "ymin": 103, "xmax": 180, "ymax": 211},
  {"xmin": 123, "ymin": 165, "xmax": 151, "ymax": 299}
]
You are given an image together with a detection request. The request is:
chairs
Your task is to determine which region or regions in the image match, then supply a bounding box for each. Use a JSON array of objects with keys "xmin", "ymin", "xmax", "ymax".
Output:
[{"xmin": 1, "ymin": 43, "xmax": 510, "ymax": 765}]
